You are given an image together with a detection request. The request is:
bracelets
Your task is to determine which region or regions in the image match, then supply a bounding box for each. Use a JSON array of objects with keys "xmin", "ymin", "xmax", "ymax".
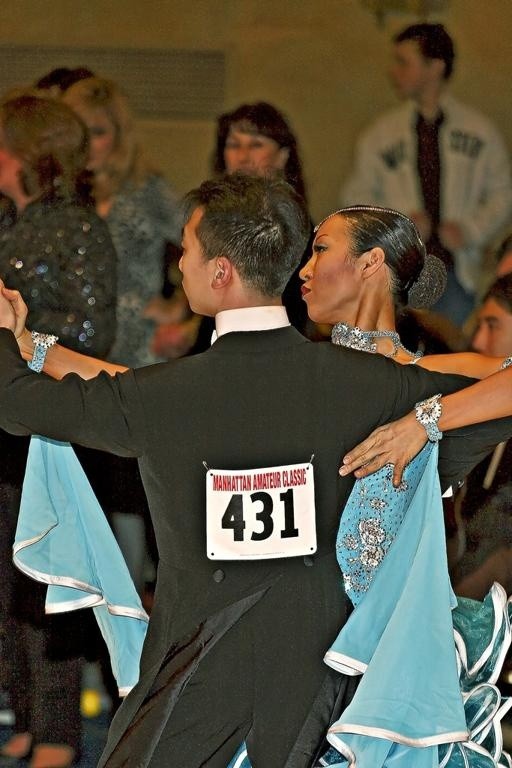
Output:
[
  {"xmin": 27, "ymin": 325, "xmax": 59, "ymax": 378},
  {"xmin": 413, "ymin": 393, "xmax": 448, "ymax": 445}
]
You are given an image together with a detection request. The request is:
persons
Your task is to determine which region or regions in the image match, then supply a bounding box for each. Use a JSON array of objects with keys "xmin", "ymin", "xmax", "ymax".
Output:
[
  {"xmin": 31, "ymin": 62, "xmax": 94, "ymax": 97},
  {"xmin": 58, "ymin": 75, "xmax": 194, "ymax": 720},
  {"xmin": 436, "ymin": 273, "xmax": 512, "ymax": 605},
  {"xmin": 154, "ymin": 97, "xmax": 318, "ymax": 357},
  {"xmin": 337, "ymin": 18, "xmax": 511, "ymax": 332},
  {"xmin": 0, "ymin": 97, "xmax": 119, "ymax": 768}
]
